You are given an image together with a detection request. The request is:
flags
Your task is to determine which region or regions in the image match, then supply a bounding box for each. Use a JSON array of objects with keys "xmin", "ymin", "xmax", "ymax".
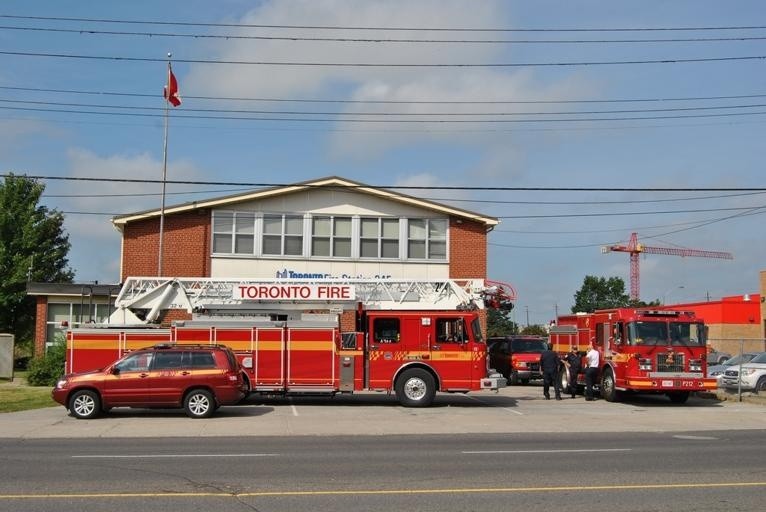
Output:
[{"xmin": 163, "ymin": 63, "xmax": 182, "ymax": 107}]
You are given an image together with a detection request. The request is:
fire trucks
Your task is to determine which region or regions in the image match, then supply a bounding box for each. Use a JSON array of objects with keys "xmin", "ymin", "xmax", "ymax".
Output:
[
  {"xmin": 65, "ymin": 276, "xmax": 516, "ymax": 407},
  {"xmin": 548, "ymin": 308, "xmax": 718, "ymax": 404}
]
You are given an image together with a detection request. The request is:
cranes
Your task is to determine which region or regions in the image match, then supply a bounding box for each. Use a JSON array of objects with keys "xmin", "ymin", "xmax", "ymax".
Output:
[{"xmin": 601, "ymin": 233, "xmax": 734, "ymax": 300}]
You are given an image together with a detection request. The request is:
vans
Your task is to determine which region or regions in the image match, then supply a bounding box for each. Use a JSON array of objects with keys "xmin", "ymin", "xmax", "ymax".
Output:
[{"xmin": 489, "ymin": 336, "xmax": 547, "ymax": 386}]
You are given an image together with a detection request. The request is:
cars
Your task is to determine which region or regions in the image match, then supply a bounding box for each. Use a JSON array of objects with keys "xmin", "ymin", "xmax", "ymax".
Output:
[
  {"xmin": 707, "ymin": 352, "xmax": 766, "ymax": 393},
  {"xmin": 51, "ymin": 344, "xmax": 248, "ymax": 419}
]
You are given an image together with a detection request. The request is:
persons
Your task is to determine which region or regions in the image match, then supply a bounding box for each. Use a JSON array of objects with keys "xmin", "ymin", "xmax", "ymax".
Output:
[
  {"xmin": 561, "ymin": 345, "xmax": 599, "ymax": 401},
  {"xmin": 539, "ymin": 343, "xmax": 562, "ymax": 400}
]
